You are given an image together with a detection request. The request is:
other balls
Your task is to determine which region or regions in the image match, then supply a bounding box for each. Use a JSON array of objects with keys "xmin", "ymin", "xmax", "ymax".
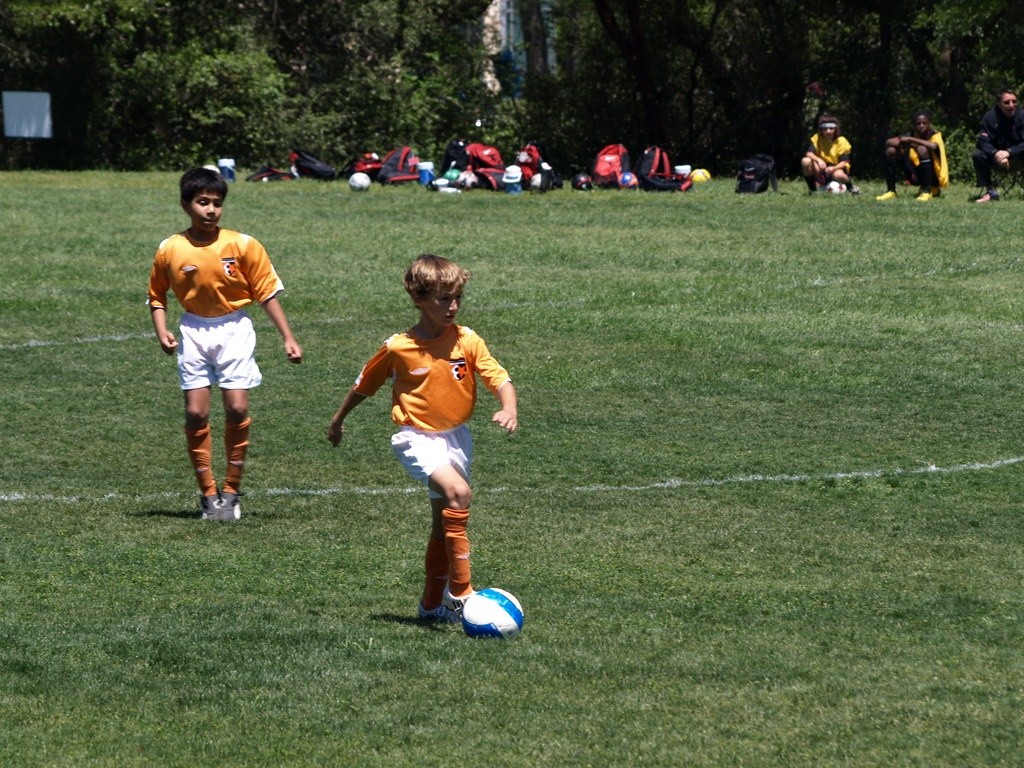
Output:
[
  {"xmin": 827, "ymin": 180, "xmax": 847, "ymax": 194},
  {"xmin": 616, "ymin": 172, "xmax": 638, "ymax": 190},
  {"xmin": 571, "ymin": 173, "xmax": 592, "ymax": 191},
  {"xmin": 690, "ymin": 168, "xmax": 711, "ymax": 182},
  {"xmin": 444, "ymin": 169, "xmax": 478, "ymax": 190},
  {"xmin": 349, "ymin": 172, "xmax": 372, "ymax": 191}
]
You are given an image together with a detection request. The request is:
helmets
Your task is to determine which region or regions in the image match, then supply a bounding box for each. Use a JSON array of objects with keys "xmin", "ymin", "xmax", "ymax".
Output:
[
  {"xmin": 617, "ymin": 172, "xmax": 639, "ymax": 190},
  {"xmin": 505, "ymin": 183, "xmax": 521, "ymax": 194},
  {"xmin": 530, "ymin": 173, "xmax": 552, "ymax": 189},
  {"xmin": 690, "ymin": 169, "xmax": 711, "ymax": 185},
  {"xmin": 572, "ymin": 173, "xmax": 592, "ymax": 191}
]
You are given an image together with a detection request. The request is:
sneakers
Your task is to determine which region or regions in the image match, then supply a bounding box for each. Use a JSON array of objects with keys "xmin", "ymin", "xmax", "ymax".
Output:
[
  {"xmin": 200, "ymin": 489, "xmax": 222, "ymax": 523},
  {"xmin": 975, "ymin": 191, "xmax": 996, "ymax": 204},
  {"xmin": 443, "ymin": 586, "xmax": 477, "ymax": 614},
  {"xmin": 218, "ymin": 492, "xmax": 244, "ymax": 525},
  {"xmin": 916, "ymin": 193, "xmax": 933, "ymax": 202},
  {"xmin": 418, "ymin": 602, "xmax": 463, "ymax": 625},
  {"xmin": 877, "ymin": 191, "xmax": 896, "ymax": 201}
]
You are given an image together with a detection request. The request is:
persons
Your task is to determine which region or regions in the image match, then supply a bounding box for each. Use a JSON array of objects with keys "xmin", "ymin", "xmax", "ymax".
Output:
[
  {"xmin": 876, "ymin": 112, "xmax": 949, "ymax": 200},
  {"xmin": 327, "ymin": 255, "xmax": 518, "ymax": 621},
  {"xmin": 801, "ymin": 114, "xmax": 860, "ymax": 194},
  {"xmin": 145, "ymin": 167, "xmax": 303, "ymax": 520},
  {"xmin": 972, "ymin": 90, "xmax": 1024, "ymax": 202}
]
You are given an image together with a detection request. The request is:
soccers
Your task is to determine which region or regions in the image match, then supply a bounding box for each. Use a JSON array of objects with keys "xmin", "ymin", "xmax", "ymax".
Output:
[{"xmin": 460, "ymin": 587, "xmax": 525, "ymax": 640}]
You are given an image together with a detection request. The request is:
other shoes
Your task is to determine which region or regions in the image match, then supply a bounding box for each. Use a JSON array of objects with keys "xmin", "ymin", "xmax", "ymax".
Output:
[{"xmin": 850, "ymin": 186, "xmax": 859, "ymax": 193}]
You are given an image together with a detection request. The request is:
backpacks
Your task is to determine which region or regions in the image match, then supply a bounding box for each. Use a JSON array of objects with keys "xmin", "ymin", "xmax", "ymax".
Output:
[
  {"xmin": 634, "ymin": 145, "xmax": 676, "ymax": 191},
  {"xmin": 594, "ymin": 144, "xmax": 631, "ymax": 189},
  {"xmin": 732, "ymin": 152, "xmax": 778, "ymax": 194},
  {"xmin": 244, "ymin": 139, "xmax": 563, "ymax": 195}
]
